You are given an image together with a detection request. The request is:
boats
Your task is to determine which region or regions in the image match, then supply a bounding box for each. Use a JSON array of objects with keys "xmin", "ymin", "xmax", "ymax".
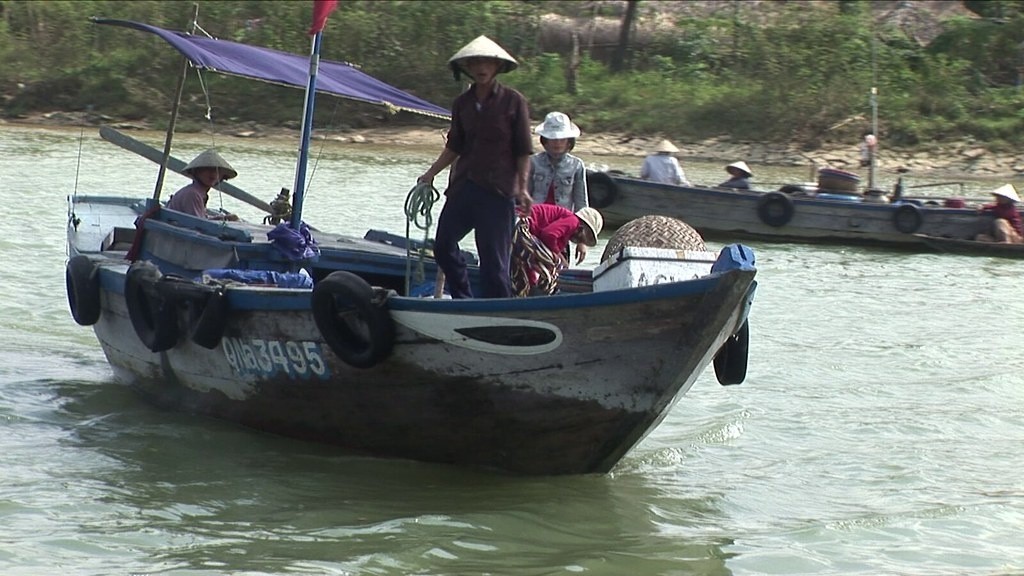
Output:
[
  {"xmin": 585, "ymin": 166, "xmax": 1024, "ymax": 253},
  {"xmin": 63, "ymin": 192, "xmax": 758, "ymax": 479}
]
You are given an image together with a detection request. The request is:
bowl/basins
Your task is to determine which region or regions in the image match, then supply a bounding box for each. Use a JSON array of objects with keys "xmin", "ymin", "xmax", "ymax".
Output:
[
  {"xmin": 555, "ymin": 268, "xmax": 593, "ymax": 295},
  {"xmin": 817, "ymin": 168, "xmax": 860, "ymax": 191}
]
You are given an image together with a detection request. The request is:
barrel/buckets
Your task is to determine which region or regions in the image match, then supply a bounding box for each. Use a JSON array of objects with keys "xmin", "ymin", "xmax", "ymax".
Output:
[
  {"xmin": 815, "ymin": 193, "xmax": 863, "ymax": 202},
  {"xmin": 948, "ymin": 198, "xmax": 963, "ymax": 208}
]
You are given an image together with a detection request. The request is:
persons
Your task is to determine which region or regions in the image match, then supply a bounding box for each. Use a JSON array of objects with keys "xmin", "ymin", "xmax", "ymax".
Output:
[
  {"xmin": 718, "ymin": 161, "xmax": 753, "ymax": 189},
  {"xmin": 975, "ymin": 183, "xmax": 1024, "ymax": 245},
  {"xmin": 417, "ymin": 36, "xmax": 536, "ymax": 299},
  {"xmin": 164, "ymin": 148, "xmax": 239, "ymax": 222},
  {"xmin": 641, "ymin": 140, "xmax": 693, "ymax": 188},
  {"xmin": 510, "ymin": 110, "xmax": 603, "ymax": 299}
]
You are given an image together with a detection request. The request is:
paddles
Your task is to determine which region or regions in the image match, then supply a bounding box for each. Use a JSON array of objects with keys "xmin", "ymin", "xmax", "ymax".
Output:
[
  {"xmin": 99, "ymin": 126, "xmax": 319, "ymax": 232},
  {"xmin": 908, "ymin": 182, "xmax": 965, "ymax": 188}
]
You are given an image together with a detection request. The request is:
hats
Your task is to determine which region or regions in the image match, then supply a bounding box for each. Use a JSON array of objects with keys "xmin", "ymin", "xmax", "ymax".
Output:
[
  {"xmin": 450, "ymin": 34, "xmax": 517, "ymax": 73},
  {"xmin": 182, "ymin": 150, "xmax": 237, "ymax": 180},
  {"xmin": 534, "ymin": 111, "xmax": 580, "ymax": 139},
  {"xmin": 654, "ymin": 139, "xmax": 680, "ymax": 152},
  {"xmin": 727, "ymin": 161, "xmax": 753, "ymax": 178},
  {"xmin": 576, "ymin": 205, "xmax": 603, "ymax": 247},
  {"xmin": 991, "ymin": 184, "xmax": 1022, "ymax": 202}
]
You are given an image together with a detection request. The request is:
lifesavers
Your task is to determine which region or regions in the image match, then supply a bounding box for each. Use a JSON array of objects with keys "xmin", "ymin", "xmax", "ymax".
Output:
[
  {"xmin": 66, "ymin": 255, "xmax": 102, "ymax": 325},
  {"xmin": 311, "ymin": 271, "xmax": 396, "ymax": 368},
  {"xmin": 894, "ymin": 204, "xmax": 922, "ymax": 233},
  {"xmin": 125, "ymin": 261, "xmax": 180, "ymax": 352},
  {"xmin": 779, "ymin": 185, "xmax": 807, "ymax": 195},
  {"xmin": 586, "ymin": 172, "xmax": 616, "ymax": 209},
  {"xmin": 714, "ymin": 317, "xmax": 749, "ymax": 385},
  {"xmin": 189, "ymin": 295, "xmax": 231, "ymax": 349},
  {"xmin": 758, "ymin": 192, "xmax": 793, "ymax": 227}
]
